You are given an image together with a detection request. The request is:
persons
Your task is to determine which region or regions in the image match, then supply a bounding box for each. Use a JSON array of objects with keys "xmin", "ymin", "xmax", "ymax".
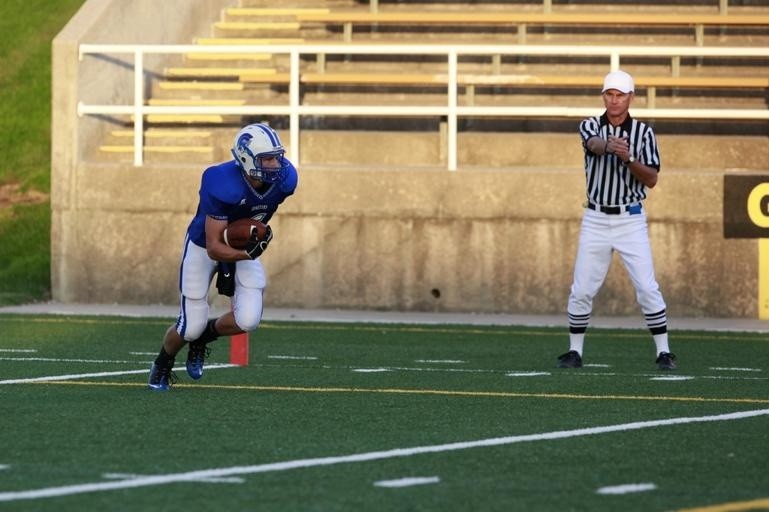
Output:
[
  {"xmin": 554, "ymin": 70, "xmax": 677, "ymax": 370},
  {"xmin": 148, "ymin": 123, "xmax": 297, "ymax": 391}
]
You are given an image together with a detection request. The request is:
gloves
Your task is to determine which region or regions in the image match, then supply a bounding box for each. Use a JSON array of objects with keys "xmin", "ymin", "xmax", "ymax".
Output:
[{"xmin": 244, "ymin": 224, "xmax": 274, "ymax": 261}]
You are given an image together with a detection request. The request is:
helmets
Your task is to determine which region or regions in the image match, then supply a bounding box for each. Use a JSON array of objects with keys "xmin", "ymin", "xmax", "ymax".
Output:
[{"xmin": 231, "ymin": 123, "xmax": 287, "ymax": 181}]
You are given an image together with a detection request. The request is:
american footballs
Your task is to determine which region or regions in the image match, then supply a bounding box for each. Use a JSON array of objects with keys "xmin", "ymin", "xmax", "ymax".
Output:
[{"xmin": 220, "ymin": 219, "xmax": 267, "ymax": 250}]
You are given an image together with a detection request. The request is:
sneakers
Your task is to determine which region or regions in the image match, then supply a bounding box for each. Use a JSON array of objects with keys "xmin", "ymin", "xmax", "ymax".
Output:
[
  {"xmin": 656, "ymin": 351, "xmax": 676, "ymax": 371},
  {"xmin": 555, "ymin": 351, "xmax": 583, "ymax": 368},
  {"xmin": 186, "ymin": 342, "xmax": 211, "ymax": 380},
  {"xmin": 148, "ymin": 358, "xmax": 175, "ymax": 393}
]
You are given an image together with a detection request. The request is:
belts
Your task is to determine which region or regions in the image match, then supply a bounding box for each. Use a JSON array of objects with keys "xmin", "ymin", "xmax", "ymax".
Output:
[{"xmin": 587, "ymin": 202, "xmax": 642, "ymax": 214}]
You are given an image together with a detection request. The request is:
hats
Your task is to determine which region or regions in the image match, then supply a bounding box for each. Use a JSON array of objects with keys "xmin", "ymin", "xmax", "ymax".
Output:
[{"xmin": 601, "ymin": 70, "xmax": 634, "ymax": 93}]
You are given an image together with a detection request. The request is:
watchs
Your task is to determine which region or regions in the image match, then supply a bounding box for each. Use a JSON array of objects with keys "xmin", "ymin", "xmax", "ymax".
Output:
[{"xmin": 624, "ymin": 156, "xmax": 635, "ymax": 165}]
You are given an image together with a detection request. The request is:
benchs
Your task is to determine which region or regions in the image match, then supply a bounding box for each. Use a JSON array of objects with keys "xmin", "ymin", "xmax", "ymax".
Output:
[{"xmin": 239, "ymin": 0, "xmax": 769, "ymax": 106}]
